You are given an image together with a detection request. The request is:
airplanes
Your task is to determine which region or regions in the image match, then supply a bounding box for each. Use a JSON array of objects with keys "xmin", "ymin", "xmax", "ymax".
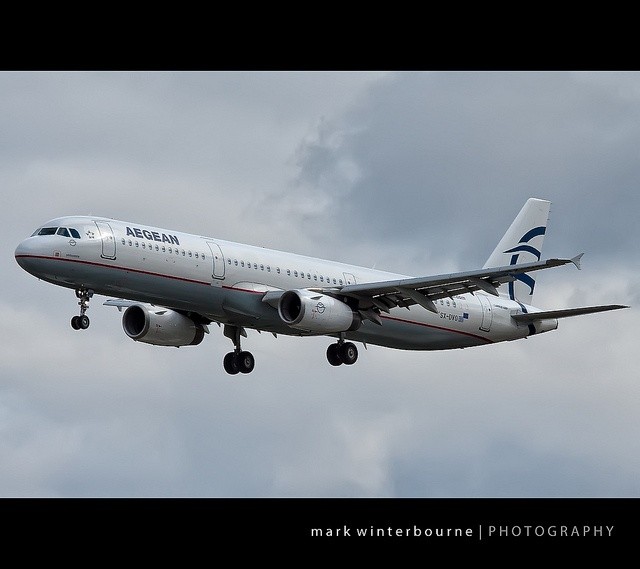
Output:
[{"xmin": 14, "ymin": 197, "xmax": 631, "ymax": 377}]
